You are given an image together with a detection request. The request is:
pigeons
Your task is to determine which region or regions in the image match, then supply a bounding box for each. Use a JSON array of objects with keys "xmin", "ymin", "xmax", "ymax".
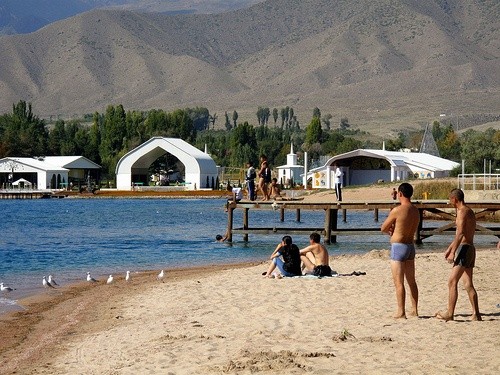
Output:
[
  {"xmin": 86, "ymin": 272, "xmax": 100, "ymax": 287},
  {"xmin": 106, "ymin": 274, "xmax": 114, "ymax": 286},
  {"xmin": 125, "ymin": 270, "xmax": 132, "ymax": 283},
  {"xmin": 43, "ymin": 275, "xmax": 60, "ymax": 289},
  {"xmin": 0, "ymin": 282, "xmax": 17, "ymax": 298},
  {"xmin": 157, "ymin": 270, "xmax": 165, "ymax": 282}
]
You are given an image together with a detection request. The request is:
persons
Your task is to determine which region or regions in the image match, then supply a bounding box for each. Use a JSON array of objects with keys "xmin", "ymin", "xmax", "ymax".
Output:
[
  {"xmin": 215, "ymin": 233, "xmax": 228, "ymax": 242},
  {"xmin": 334, "ymin": 163, "xmax": 344, "ymax": 201},
  {"xmin": 245, "ymin": 161, "xmax": 256, "ymax": 201},
  {"xmin": 436, "ymin": 188, "xmax": 482, "ymax": 321},
  {"xmin": 390, "ymin": 188, "xmax": 397, "ymax": 200},
  {"xmin": 226, "ymin": 186, "xmax": 242, "ymax": 212},
  {"xmin": 381, "ymin": 183, "xmax": 420, "ymax": 320},
  {"xmin": 268, "ymin": 178, "xmax": 304, "ymax": 200},
  {"xmin": 263, "ymin": 236, "xmax": 302, "ymax": 278},
  {"xmin": 299, "ymin": 232, "xmax": 331, "ymax": 275},
  {"xmin": 257, "ymin": 155, "xmax": 271, "ymax": 201}
]
